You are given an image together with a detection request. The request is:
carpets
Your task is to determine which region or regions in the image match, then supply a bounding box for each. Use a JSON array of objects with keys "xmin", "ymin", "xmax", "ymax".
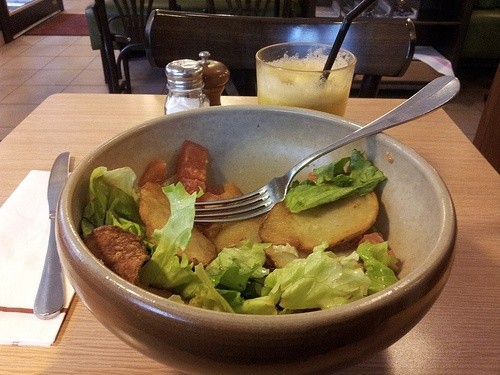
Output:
[{"xmin": 25, "ymin": 13, "xmax": 89, "ymax": 36}]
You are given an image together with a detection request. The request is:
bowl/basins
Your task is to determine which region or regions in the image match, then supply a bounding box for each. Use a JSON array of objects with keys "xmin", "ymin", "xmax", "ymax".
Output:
[{"xmin": 54, "ymin": 104, "xmax": 458, "ymax": 375}]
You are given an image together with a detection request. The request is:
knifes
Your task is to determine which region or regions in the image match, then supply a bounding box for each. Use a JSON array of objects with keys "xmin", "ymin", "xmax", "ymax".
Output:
[{"xmin": 32, "ymin": 151, "xmax": 72, "ymax": 320}]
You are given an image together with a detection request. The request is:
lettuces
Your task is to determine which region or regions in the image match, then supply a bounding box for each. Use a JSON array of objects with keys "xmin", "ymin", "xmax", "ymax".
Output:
[{"xmin": 81, "ymin": 149, "xmax": 401, "ymax": 315}]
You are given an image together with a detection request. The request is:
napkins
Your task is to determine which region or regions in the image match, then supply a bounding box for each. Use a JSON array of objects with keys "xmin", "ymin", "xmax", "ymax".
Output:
[{"xmin": 0, "ymin": 169, "xmax": 78, "ymax": 348}]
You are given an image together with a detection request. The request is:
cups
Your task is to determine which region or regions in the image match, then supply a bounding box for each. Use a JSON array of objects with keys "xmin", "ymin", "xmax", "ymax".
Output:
[{"xmin": 256, "ymin": 41, "xmax": 358, "ymax": 117}]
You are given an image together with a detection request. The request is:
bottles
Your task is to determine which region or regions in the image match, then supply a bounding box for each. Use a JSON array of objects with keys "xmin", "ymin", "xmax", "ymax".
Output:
[{"xmin": 164, "ymin": 58, "xmax": 211, "ymax": 117}]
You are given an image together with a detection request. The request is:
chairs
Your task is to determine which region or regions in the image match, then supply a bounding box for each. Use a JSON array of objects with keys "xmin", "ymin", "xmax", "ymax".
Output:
[
  {"xmin": 205, "ymin": 0, "xmax": 315, "ymax": 95},
  {"xmin": 144, "ymin": 8, "xmax": 418, "ymax": 104},
  {"xmin": 93, "ymin": 0, "xmax": 180, "ymax": 94}
]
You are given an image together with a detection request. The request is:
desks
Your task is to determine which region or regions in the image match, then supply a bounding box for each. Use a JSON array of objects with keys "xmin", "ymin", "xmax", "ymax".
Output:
[{"xmin": 87, "ymin": 0, "xmax": 317, "ymax": 51}]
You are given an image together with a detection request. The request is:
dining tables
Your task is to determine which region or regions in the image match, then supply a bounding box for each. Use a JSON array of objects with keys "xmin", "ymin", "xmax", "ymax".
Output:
[{"xmin": 0, "ymin": 92, "xmax": 500, "ymax": 375}]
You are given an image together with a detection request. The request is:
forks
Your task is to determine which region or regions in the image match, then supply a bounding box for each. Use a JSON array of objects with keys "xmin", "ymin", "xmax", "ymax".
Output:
[{"xmin": 192, "ymin": 76, "xmax": 460, "ymax": 223}]
